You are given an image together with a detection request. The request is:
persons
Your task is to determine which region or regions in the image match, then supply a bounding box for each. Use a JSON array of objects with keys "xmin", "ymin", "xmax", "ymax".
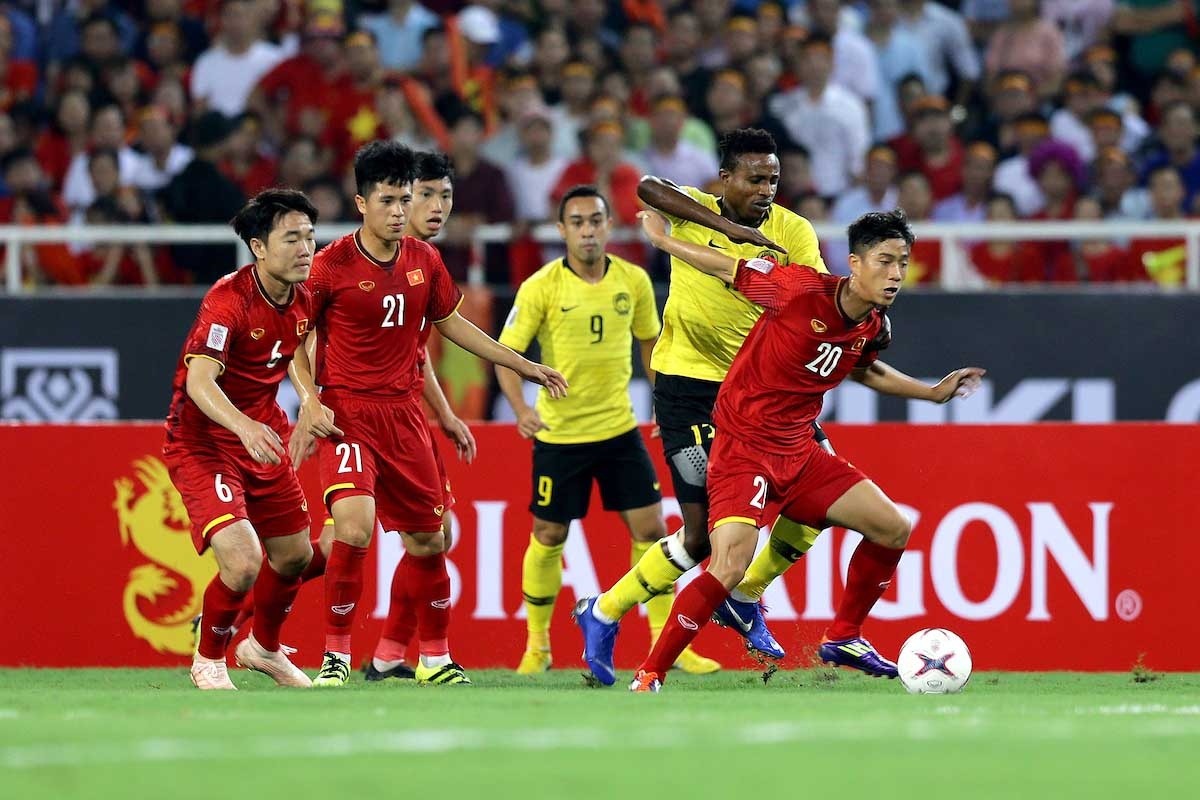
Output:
[
  {"xmin": 496, "ymin": 185, "xmax": 721, "ymax": 679},
  {"xmin": 565, "ymin": 127, "xmax": 838, "ymax": 684},
  {"xmin": 2, "ymin": 0, "xmax": 1200, "ymax": 287},
  {"xmin": 626, "ymin": 205, "xmax": 988, "ymax": 694},
  {"xmin": 191, "ymin": 151, "xmax": 569, "ymax": 682},
  {"xmin": 286, "ymin": 138, "xmax": 569, "ymax": 688},
  {"xmin": 161, "ymin": 186, "xmax": 315, "ymax": 687}
]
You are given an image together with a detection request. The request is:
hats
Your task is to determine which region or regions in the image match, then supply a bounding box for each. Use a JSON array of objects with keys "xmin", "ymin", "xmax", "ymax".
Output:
[
  {"xmin": 457, "ymin": 5, "xmax": 502, "ymax": 44},
  {"xmin": 305, "ymin": 0, "xmax": 343, "ymax": 38}
]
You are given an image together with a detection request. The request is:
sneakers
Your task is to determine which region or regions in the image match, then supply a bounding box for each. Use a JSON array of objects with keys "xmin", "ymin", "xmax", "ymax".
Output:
[
  {"xmin": 648, "ymin": 643, "xmax": 720, "ymax": 674},
  {"xmin": 312, "ymin": 652, "xmax": 351, "ymax": 687},
  {"xmin": 570, "ymin": 595, "xmax": 619, "ymax": 687},
  {"xmin": 710, "ymin": 593, "xmax": 785, "ymax": 661},
  {"xmin": 517, "ymin": 650, "xmax": 552, "ymax": 674},
  {"xmin": 234, "ymin": 626, "xmax": 313, "ymax": 688},
  {"xmin": 188, "ymin": 650, "xmax": 239, "ymax": 690},
  {"xmin": 629, "ymin": 669, "xmax": 662, "ymax": 694},
  {"xmin": 415, "ymin": 655, "xmax": 472, "ymax": 685},
  {"xmin": 817, "ymin": 627, "xmax": 898, "ymax": 679},
  {"xmin": 364, "ymin": 658, "xmax": 415, "ymax": 683}
]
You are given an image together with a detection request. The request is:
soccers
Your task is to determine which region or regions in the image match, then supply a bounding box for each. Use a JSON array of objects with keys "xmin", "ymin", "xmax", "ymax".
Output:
[{"xmin": 898, "ymin": 626, "xmax": 972, "ymax": 698}]
